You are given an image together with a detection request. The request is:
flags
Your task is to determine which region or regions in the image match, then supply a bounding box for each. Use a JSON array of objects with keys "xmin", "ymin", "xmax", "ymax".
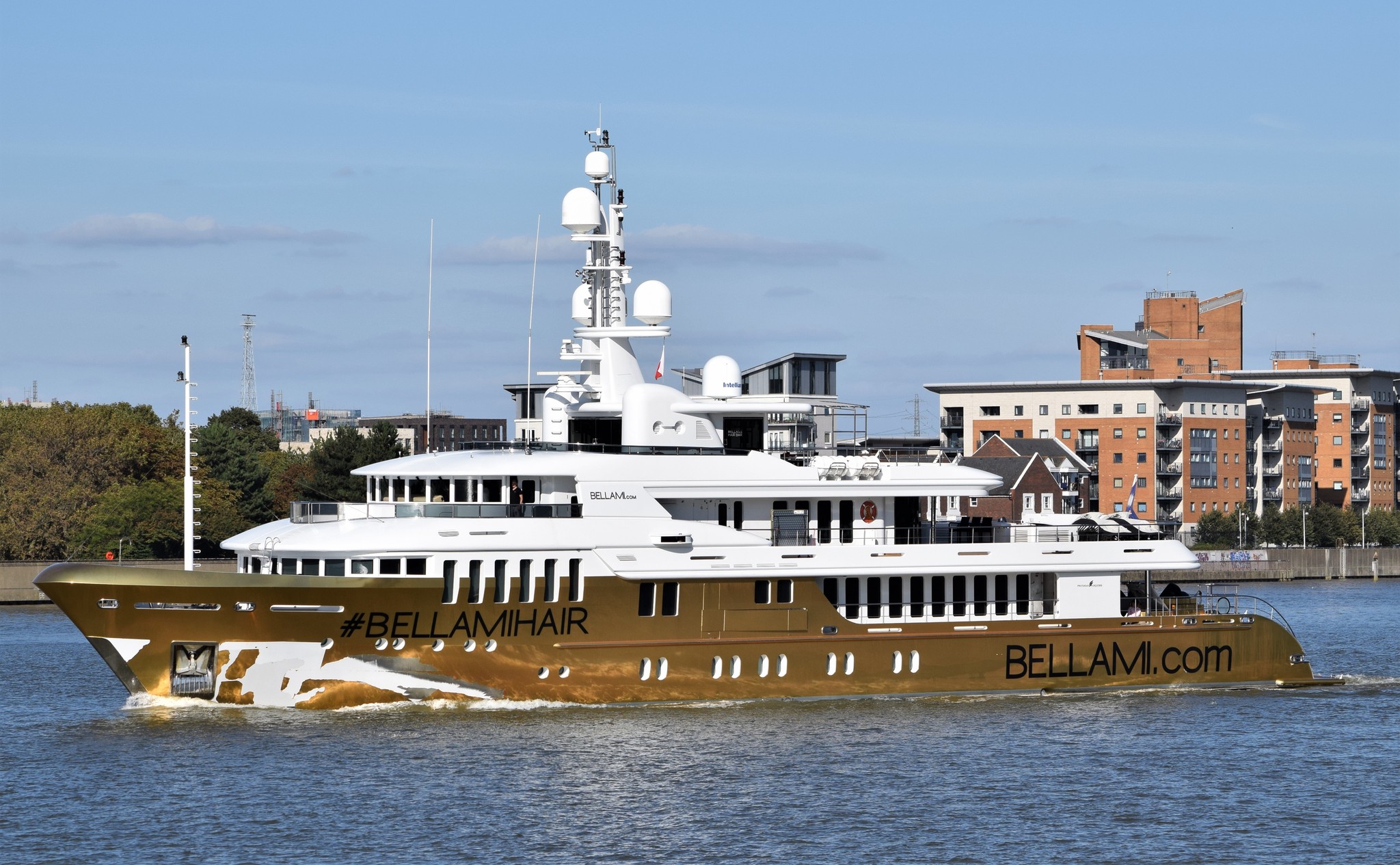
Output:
[{"xmin": 655, "ymin": 345, "xmax": 664, "ymax": 380}]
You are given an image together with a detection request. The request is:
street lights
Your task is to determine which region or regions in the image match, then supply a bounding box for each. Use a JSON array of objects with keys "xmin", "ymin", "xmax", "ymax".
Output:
[
  {"xmin": 1238, "ymin": 509, "xmax": 1244, "ymax": 549},
  {"xmin": 1302, "ymin": 508, "xmax": 1309, "ymax": 549},
  {"xmin": 1362, "ymin": 508, "xmax": 1368, "ymax": 548},
  {"xmin": 1244, "ymin": 514, "xmax": 1249, "ymax": 547}
]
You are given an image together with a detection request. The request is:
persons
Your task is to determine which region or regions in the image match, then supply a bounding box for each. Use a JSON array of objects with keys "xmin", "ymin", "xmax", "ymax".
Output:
[
  {"xmin": 510, "ymin": 479, "xmax": 523, "ymax": 517},
  {"xmin": 1123, "ymin": 600, "xmax": 1141, "ymax": 624}
]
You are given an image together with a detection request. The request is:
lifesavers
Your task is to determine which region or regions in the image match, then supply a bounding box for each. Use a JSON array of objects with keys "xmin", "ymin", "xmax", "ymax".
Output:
[{"xmin": 860, "ymin": 501, "xmax": 878, "ymax": 523}]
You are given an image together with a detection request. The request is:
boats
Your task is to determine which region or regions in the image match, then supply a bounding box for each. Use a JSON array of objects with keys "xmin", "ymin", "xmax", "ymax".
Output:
[{"xmin": 31, "ymin": 124, "xmax": 1322, "ymax": 723}]
